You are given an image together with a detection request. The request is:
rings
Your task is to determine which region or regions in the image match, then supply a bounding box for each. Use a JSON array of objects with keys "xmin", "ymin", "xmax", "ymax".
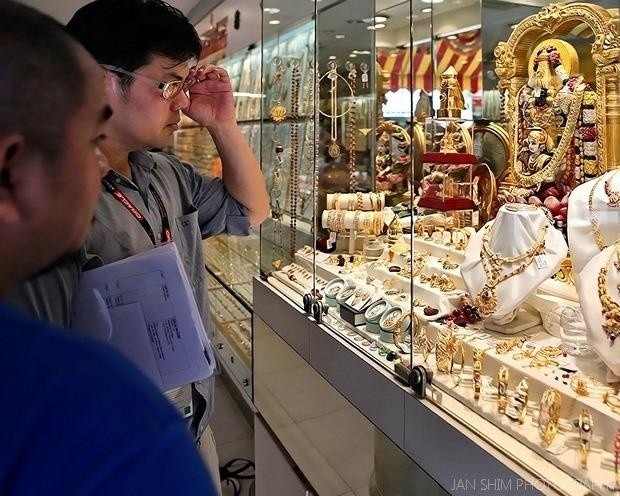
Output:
[{"xmin": 203, "ymin": 238, "xmax": 259, "ymax": 357}]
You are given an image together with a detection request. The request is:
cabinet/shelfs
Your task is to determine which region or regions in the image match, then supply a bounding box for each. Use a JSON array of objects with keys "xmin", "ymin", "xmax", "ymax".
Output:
[{"xmin": 165, "ymin": 1, "xmax": 619, "ymax": 496}]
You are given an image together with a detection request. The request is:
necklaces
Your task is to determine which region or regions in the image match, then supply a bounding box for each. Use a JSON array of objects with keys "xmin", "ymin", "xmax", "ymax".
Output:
[
  {"xmin": 510, "ymin": 81, "xmax": 586, "ymax": 188},
  {"xmin": 588, "ymin": 172, "xmax": 608, "ymax": 253},
  {"xmin": 473, "ymin": 216, "xmax": 550, "ymax": 315},
  {"xmin": 268, "ymin": 62, "xmax": 374, "ymax": 270},
  {"xmin": 597, "ymin": 238, "xmax": 620, "ymax": 342},
  {"xmin": 605, "ymin": 170, "xmax": 620, "ymax": 207}
]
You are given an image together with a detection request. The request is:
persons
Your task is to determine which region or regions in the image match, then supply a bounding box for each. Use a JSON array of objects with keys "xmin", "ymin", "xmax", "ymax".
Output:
[
  {"xmin": 516, "ymin": 125, "xmax": 556, "ymax": 196},
  {"xmin": 1, "ymin": 0, "xmax": 220, "ymax": 495},
  {"xmin": 375, "ymin": 133, "xmax": 394, "ymax": 179},
  {"xmin": 15, "ymin": 0, "xmax": 272, "ymax": 495}
]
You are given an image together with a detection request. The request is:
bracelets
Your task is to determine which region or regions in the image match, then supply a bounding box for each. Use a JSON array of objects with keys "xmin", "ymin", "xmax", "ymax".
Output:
[
  {"xmin": 391, "ymin": 312, "xmax": 619, "ymax": 481},
  {"xmin": 326, "ymin": 192, "xmax": 384, "ymax": 237}
]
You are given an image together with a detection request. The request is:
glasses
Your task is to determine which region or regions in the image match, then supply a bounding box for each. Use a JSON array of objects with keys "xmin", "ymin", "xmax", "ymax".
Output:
[{"xmin": 98, "ymin": 59, "xmax": 193, "ymax": 102}]
